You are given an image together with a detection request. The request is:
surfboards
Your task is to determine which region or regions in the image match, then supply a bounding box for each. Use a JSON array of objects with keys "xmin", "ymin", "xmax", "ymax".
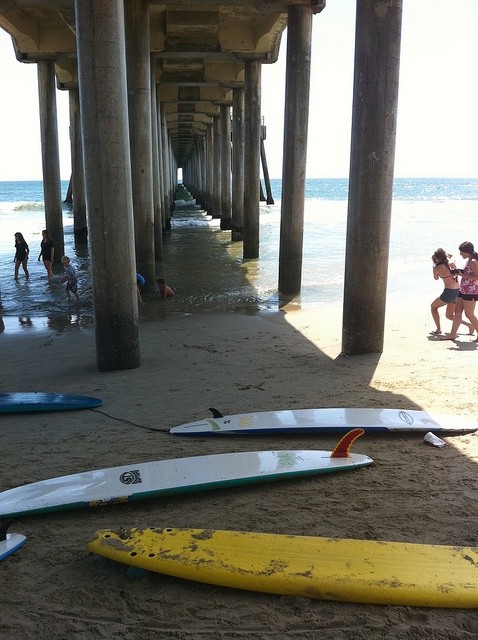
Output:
[
  {"xmin": 0, "ymin": 429, "xmax": 373, "ymax": 516},
  {"xmin": 169, "ymin": 408, "xmax": 478, "ymax": 438},
  {"xmin": 0, "ymin": 517, "xmax": 28, "ymax": 562},
  {"xmin": 0, "ymin": 390, "xmax": 104, "ymax": 414},
  {"xmin": 85, "ymin": 526, "xmax": 477, "ymax": 610}
]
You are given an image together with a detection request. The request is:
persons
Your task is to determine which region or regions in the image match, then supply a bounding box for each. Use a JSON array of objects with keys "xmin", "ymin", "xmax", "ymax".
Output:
[
  {"xmin": 14, "ymin": 232, "xmax": 29, "ymax": 280},
  {"xmin": 446, "ymin": 252, "xmax": 460, "ymax": 286},
  {"xmin": 429, "ymin": 247, "xmax": 475, "ymax": 336},
  {"xmin": 156, "ymin": 279, "xmax": 175, "ymax": 298},
  {"xmin": 38, "ymin": 230, "xmax": 55, "ymax": 279},
  {"xmin": 61, "ymin": 256, "xmax": 80, "ymax": 301},
  {"xmin": 136, "ymin": 272, "xmax": 145, "ymax": 305},
  {"xmin": 435, "ymin": 242, "xmax": 478, "ymax": 343}
]
